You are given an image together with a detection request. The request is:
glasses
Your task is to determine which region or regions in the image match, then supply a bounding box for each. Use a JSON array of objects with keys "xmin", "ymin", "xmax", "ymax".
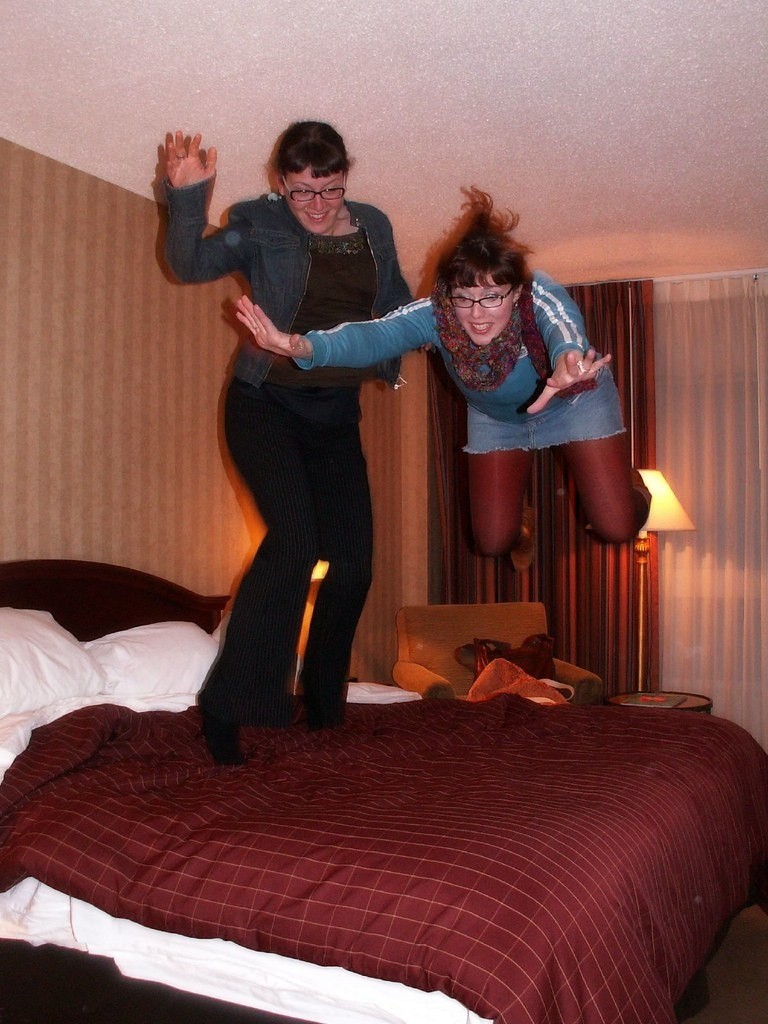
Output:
[
  {"xmin": 448, "ymin": 285, "xmax": 517, "ymax": 308},
  {"xmin": 283, "ymin": 171, "xmax": 347, "ymax": 202}
]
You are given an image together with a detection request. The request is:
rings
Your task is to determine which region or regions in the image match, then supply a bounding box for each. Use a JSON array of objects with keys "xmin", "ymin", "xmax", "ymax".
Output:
[
  {"xmin": 176, "ymin": 154, "xmax": 185, "ymax": 160},
  {"xmin": 254, "ymin": 327, "xmax": 259, "ymax": 330},
  {"xmin": 576, "ymin": 360, "xmax": 592, "ymax": 375}
]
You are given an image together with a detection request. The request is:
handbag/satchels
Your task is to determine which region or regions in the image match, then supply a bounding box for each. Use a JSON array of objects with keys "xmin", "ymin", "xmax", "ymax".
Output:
[{"xmin": 453, "ymin": 634, "xmax": 556, "ymax": 683}]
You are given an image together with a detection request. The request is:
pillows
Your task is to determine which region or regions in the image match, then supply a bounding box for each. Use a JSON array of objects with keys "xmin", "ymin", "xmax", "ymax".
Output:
[{"xmin": 0, "ymin": 605, "xmax": 219, "ymax": 723}]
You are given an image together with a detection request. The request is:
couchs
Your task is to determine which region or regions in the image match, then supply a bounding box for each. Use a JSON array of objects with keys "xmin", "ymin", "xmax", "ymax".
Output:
[{"xmin": 391, "ymin": 602, "xmax": 604, "ymax": 704}]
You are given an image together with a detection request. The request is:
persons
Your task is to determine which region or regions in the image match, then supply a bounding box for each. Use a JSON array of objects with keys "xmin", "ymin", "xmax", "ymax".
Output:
[
  {"xmin": 236, "ymin": 186, "xmax": 652, "ymax": 576},
  {"xmin": 155, "ymin": 117, "xmax": 432, "ymax": 771}
]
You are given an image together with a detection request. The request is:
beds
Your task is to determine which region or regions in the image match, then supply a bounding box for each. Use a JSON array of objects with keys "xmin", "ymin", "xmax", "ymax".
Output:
[{"xmin": 0, "ymin": 560, "xmax": 768, "ymax": 1024}]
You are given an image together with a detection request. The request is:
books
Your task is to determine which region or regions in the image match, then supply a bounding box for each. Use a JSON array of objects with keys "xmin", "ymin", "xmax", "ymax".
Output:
[{"xmin": 620, "ymin": 693, "xmax": 688, "ymax": 708}]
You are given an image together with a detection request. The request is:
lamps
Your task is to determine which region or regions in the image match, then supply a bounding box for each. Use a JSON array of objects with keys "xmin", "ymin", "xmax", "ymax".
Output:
[{"xmin": 588, "ymin": 469, "xmax": 699, "ymax": 693}]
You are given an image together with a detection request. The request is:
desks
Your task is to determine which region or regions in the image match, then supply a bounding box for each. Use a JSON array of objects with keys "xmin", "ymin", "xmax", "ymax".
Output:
[{"xmin": 613, "ymin": 690, "xmax": 714, "ymax": 714}]
